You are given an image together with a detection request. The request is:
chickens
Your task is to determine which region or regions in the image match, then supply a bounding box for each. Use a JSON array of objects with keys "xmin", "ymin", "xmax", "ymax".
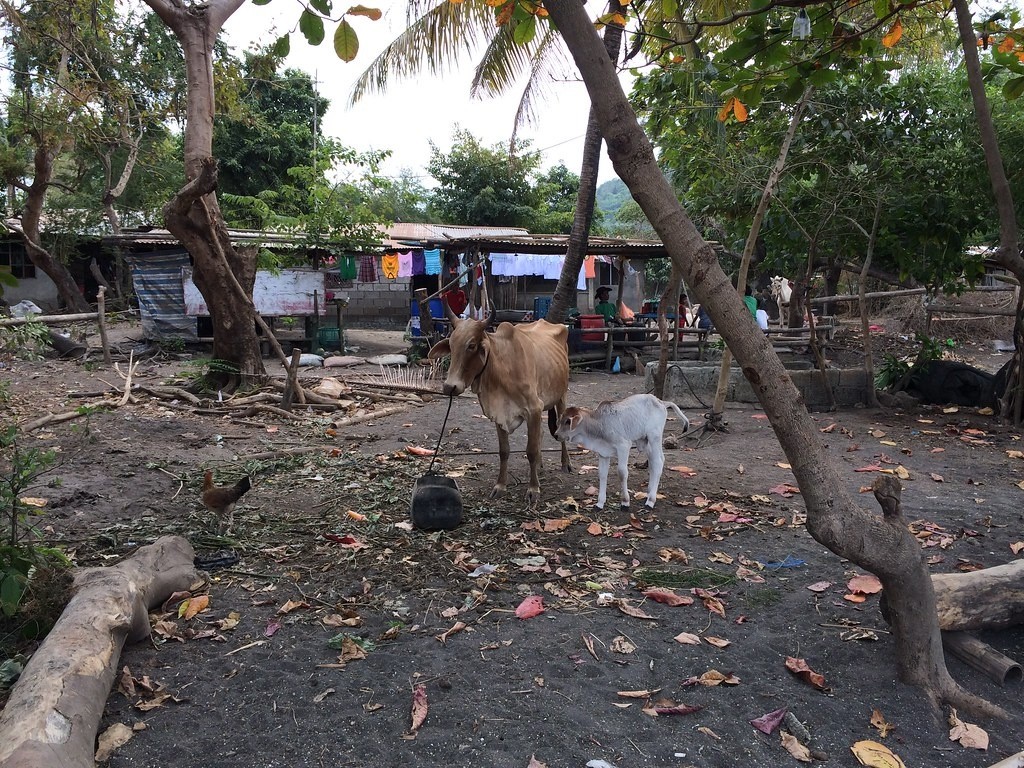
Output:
[{"xmin": 202, "ymin": 470, "xmax": 252, "ymax": 535}]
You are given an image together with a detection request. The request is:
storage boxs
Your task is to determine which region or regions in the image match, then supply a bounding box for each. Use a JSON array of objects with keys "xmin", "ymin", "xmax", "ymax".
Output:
[
  {"xmin": 318, "ymin": 326, "xmax": 346, "ymax": 346},
  {"xmin": 534, "ymin": 296, "xmax": 551, "ymax": 320}
]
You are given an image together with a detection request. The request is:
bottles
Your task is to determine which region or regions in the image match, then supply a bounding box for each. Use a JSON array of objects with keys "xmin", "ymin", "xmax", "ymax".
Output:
[{"xmin": 341, "ymin": 297, "xmax": 350, "ymax": 310}]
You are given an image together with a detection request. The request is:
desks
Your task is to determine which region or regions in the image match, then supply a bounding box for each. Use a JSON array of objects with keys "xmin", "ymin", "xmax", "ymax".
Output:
[{"xmin": 634, "ymin": 313, "xmax": 683, "ymax": 341}]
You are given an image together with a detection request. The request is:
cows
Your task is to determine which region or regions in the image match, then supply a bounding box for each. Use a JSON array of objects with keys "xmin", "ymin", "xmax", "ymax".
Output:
[
  {"xmin": 428, "ymin": 295, "xmax": 575, "ymax": 504},
  {"xmin": 553, "ymin": 393, "xmax": 689, "ymax": 511}
]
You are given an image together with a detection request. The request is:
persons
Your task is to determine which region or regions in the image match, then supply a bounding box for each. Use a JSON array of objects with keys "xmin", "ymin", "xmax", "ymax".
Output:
[
  {"xmin": 674, "ymin": 294, "xmax": 689, "ymax": 343},
  {"xmin": 441, "ymin": 280, "xmax": 467, "ymax": 318},
  {"xmin": 745, "ymin": 284, "xmax": 761, "ymax": 328},
  {"xmin": 595, "ymin": 285, "xmax": 658, "ymax": 350},
  {"xmin": 755, "ymin": 300, "xmax": 770, "ymax": 337},
  {"xmin": 690, "ymin": 302, "xmax": 715, "ymax": 342}
]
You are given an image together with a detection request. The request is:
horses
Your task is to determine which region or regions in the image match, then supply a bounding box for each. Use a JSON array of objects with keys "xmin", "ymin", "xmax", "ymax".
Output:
[{"xmin": 770, "ymin": 275, "xmax": 793, "ymax": 337}]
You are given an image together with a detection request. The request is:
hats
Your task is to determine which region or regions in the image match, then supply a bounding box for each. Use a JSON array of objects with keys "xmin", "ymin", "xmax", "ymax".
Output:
[{"xmin": 595, "ymin": 287, "xmax": 612, "ymax": 299}]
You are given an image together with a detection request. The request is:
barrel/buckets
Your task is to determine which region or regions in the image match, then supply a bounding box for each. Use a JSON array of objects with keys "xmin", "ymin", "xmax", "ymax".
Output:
[
  {"xmin": 318, "ymin": 327, "xmax": 340, "ymax": 352},
  {"xmin": 411, "ymin": 297, "xmax": 444, "ymax": 336}
]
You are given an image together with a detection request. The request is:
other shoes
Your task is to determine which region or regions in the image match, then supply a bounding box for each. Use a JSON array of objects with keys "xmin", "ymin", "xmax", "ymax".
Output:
[{"xmin": 651, "ymin": 334, "xmax": 658, "ymax": 341}]
